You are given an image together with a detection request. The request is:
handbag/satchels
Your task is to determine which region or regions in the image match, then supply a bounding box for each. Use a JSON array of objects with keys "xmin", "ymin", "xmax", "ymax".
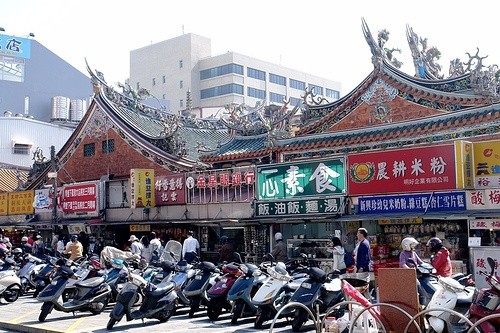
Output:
[{"xmin": 344, "ymin": 249, "xmax": 354, "ymax": 267}]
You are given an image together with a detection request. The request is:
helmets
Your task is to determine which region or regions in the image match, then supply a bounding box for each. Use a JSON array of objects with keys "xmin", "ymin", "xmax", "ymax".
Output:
[
  {"xmin": 274, "ymin": 233, "xmax": 283, "ymax": 242},
  {"xmin": 3, "ymin": 238, "xmax": 9, "ymax": 243},
  {"xmin": 426, "ymin": 237, "xmax": 442, "ymax": 252},
  {"xmin": 402, "ymin": 237, "xmax": 416, "ymax": 251},
  {"xmin": 21, "ymin": 236, "xmax": 29, "ymax": 242},
  {"xmin": 72, "ymin": 235, "xmax": 78, "ymax": 240},
  {"xmin": 220, "ymin": 236, "xmax": 229, "ymax": 242}
]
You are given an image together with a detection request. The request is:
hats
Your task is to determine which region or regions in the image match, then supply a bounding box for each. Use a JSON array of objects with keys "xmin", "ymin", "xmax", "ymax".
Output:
[
  {"xmin": 36, "ymin": 235, "xmax": 42, "ymax": 239},
  {"xmin": 128, "ymin": 235, "xmax": 138, "ymax": 241}
]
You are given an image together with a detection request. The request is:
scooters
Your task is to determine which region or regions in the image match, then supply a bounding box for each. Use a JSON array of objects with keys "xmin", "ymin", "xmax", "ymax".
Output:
[{"xmin": 1, "ymin": 241, "xmax": 500, "ymax": 333}]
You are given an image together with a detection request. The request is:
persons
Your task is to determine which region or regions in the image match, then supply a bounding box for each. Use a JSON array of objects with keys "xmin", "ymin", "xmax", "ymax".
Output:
[
  {"xmin": 218, "ymin": 236, "xmax": 233, "ymax": 263},
  {"xmin": 0, "ymin": 233, "xmax": 83, "ymax": 262},
  {"xmin": 353, "ymin": 228, "xmax": 371, "ymax": 272},
  {"xmin": 182, "ymin": 231, "xmax": 200, "ymax": 264},
  {"xmin": 327, "ymin": 237, "xmax": 347, "ymax": 276},
  {"xmin": 129, "ymin": 229, "xmax": 164, "ymax": 261},
  {"xmin": 269, "ymin": 232, "xmax": 287, "ymax": 263},
  {"xmin": 399, "ymin": 235, "xmax": 451, "ymax": 297}
]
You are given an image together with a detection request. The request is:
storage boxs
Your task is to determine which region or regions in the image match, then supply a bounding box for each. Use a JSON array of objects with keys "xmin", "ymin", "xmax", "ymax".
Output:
[
  {"xmin": 50, "ymin": 95, "xmax": 71, "ymax": 126},
  {"xmin": 68, "ymin": 98, "xmax": 87, "ymax": 129}
]
